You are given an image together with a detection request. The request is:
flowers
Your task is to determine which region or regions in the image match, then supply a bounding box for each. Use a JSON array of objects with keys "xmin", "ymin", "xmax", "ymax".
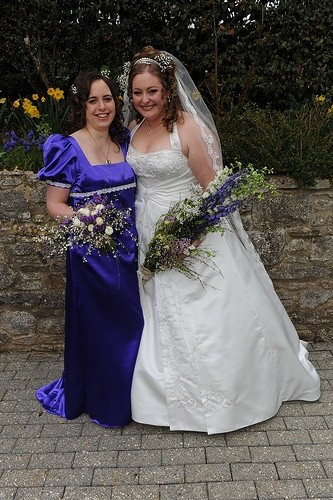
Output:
[
  {"xmin": 29, "ymin": 189, "xmax": 139, "ymax": 286},
  {"xmin": 140, "ymin": 157, "xmax": 283, "ymax": 286}
]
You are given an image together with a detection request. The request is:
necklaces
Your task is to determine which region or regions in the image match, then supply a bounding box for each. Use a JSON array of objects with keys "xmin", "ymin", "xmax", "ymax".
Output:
[
  {"xmin": 146, "ymin": 120, "xmax": 165, "ymax": 135},
  {"xmin": 85, "ymin": 127, "xmax": 111, "ymax": 164}
]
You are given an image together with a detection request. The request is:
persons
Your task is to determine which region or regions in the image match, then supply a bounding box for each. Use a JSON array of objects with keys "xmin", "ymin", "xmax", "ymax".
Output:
[
  {"xmin": 113, "ymin": 45, "xmax": 321, "ymax": 435},
  {"xmin": 35, "ymin": 71, "xmax": 144, "ymax": 428}
]
